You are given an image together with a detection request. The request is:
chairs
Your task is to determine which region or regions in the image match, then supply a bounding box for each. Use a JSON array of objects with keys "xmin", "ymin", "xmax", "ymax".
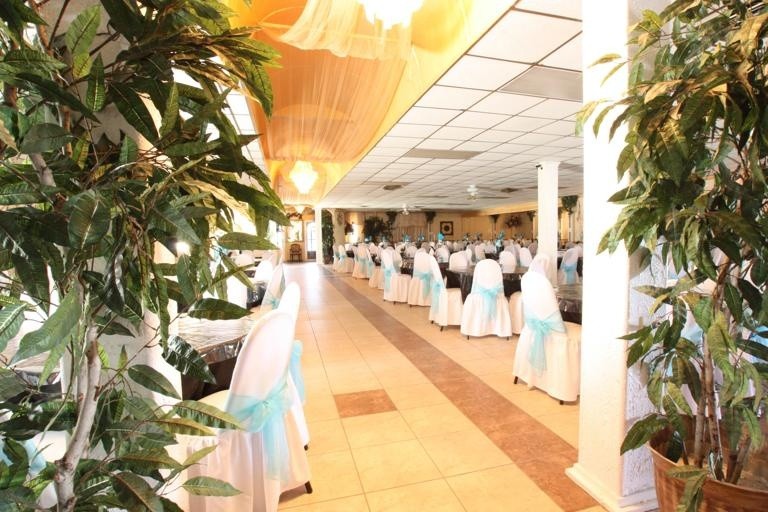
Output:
[
  {"xmin": 332, "ymin": 236, "xmax": 586, "ymax": 331},
  {"xmin": 513, "ymin": 273, "xmax": 581, "ymax": 405},
  {"xmin": 157, "ymin": 280, "xmax": 313, "ymax": 512},
  {"xmin": 509, "ymin": 253, "xmax": 556, "ymax": 335},
  {"xmin": 157, "ymin": 240, "xmax": 305, "ymax": 401},
  {"xmin": 459, "ymin": 259, "xmax": 513, "ymax": 340}
]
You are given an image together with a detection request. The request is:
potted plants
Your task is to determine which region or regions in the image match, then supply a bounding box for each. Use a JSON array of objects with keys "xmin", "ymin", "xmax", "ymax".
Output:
[{"xmin": 564, "ymin": 0, "xmax": 768, "ymax": 511}]
